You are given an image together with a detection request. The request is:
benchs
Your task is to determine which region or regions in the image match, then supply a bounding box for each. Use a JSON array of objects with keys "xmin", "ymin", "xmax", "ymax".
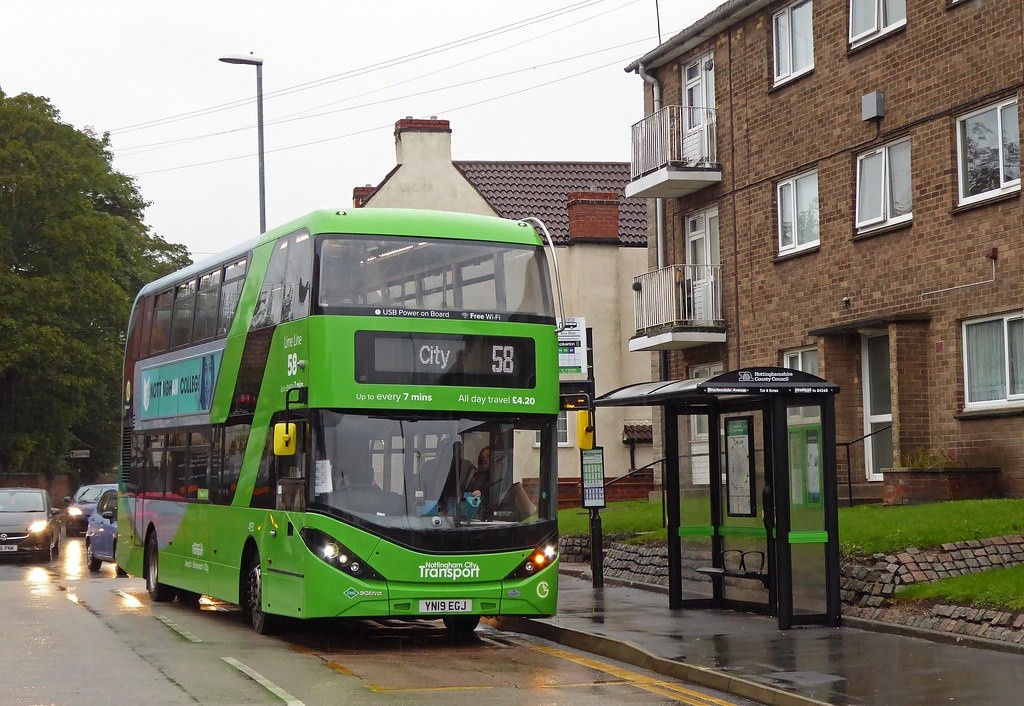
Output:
[{"xmin": 694, "ymin": 549, "xmax": 770, "ymax": 590}]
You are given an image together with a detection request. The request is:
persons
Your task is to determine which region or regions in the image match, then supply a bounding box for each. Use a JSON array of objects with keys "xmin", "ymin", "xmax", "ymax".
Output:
[{"xmin": 416, "ymin": 437, "xmax": 509, "ymax": 521}]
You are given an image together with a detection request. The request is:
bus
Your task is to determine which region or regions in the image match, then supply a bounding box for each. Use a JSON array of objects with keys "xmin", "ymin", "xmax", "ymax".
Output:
[{"xmin": 113, "ymin": 209, "xmax": 597, "ymax": 638}]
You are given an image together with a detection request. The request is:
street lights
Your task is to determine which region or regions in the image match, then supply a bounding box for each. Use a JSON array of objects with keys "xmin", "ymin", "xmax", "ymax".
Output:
[{"xmin": 219, "ymin": 54, "xmax": 270, "ymax": 234}]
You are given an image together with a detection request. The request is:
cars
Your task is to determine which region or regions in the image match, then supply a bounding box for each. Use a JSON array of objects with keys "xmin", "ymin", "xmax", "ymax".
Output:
[
  {"xmin": 0, "ymin": 486, "xmax": 64, "ymax": 562},
  {"xmin": 64, "ymin": 482, "xmax": 121, "ymax": 535},
  {"xmin": 86, "ymin": 489, "xmax": 121, "ymax": 571}
]
